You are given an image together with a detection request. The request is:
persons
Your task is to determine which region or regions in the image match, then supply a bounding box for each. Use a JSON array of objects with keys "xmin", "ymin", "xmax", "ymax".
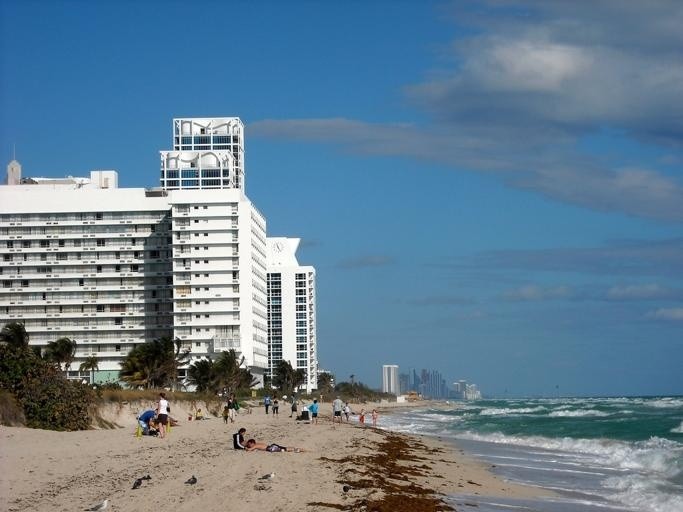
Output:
[
  {"xmin": 233, "ymin": 427, "xmax": 249, "ymax": 451},
  {"xmin": 265, "ymin": 392, "xmax": 378, "ymax": 425},
  {"xmin": 136, "ymin": 407, "xmax": 158, "ymax": 436},
  {"xmin": 243, "ymin": 439, "xmax": 305, "ymax": 453},
  {"xmin": 222, "ymin": 406, "xmax": 230, "ymax": 423},
  {"xmin": 157, "ymin": 391, "xmax": 168, "ymax": 437},
  {"xmin": 227, "ymin": 392, "xmax": 236, "ymax": 423},
  {"xmin": 195, "ymin": 408, "xmax": 203, "ymax": 421}
]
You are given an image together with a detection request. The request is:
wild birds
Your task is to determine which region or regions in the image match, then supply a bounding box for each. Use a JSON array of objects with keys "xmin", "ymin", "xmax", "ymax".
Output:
[
  {"xmin": 131, "ymin": 478, "xmax": 142, "ymax": 490},
  {"xmin": 343, "ymin": 485, "xmax": 350, "ymax": 492},
  {"xmin": 257, "ymin": 471, "xmax": 276, "ymax": 482},
  {"xmin": 84, "ymin": 497, "xmax": 111, "ymax": 512},
  {"xmin": 183, "ymin": 475, "xmax": 197, "ymax": 486}
]
